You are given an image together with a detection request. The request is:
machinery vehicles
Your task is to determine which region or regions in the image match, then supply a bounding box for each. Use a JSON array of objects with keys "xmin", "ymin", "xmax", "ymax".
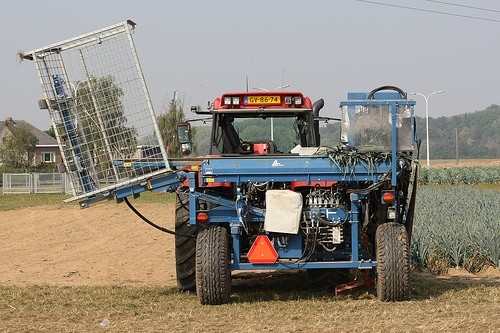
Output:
[{"xmin": 52, "ymin": 53, "xmax": 418, "ymax": 305}]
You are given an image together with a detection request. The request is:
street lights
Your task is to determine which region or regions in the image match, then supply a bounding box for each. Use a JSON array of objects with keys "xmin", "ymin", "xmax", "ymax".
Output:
[
  {"xmin": 408, "ymin": 90, "xmax": 445, "ymax": 167},
  {"xmin": 252, "ymin": 85, "xmax": 291, "ymax": 142},
  {"xmin": 61, "ymin": 79, "xmax": 94, "ymax": 130}
]
